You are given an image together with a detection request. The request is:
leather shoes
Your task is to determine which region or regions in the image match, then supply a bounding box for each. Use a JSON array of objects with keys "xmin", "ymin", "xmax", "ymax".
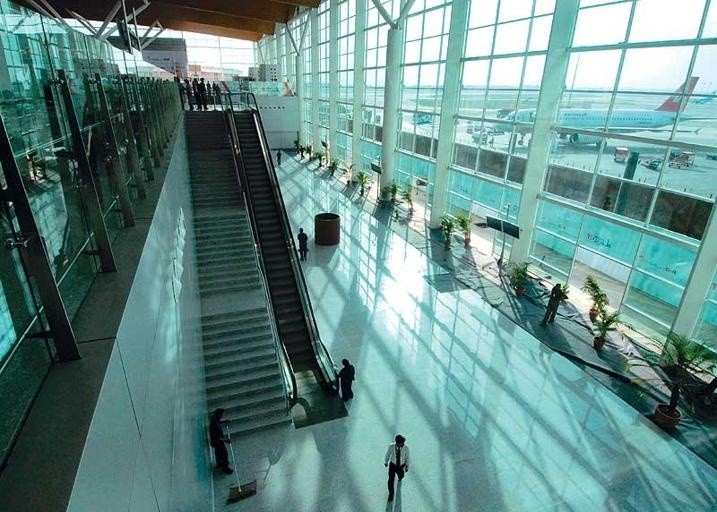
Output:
[
  {"xmin": 398, "ymin": 477, "xmax": 402, "ymax": 482},
  {"xmin": 388, "ymin": 494, "xmax": 394, "ymax": 502}
]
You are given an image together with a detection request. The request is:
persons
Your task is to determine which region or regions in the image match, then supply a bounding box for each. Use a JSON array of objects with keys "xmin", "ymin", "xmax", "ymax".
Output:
[
  {"xmin": 538, "ymin": 283, "xmax": 568, "ymax": 326},
  {"xmin": 298, "ymin": 145, "xmax": 306, "ymax": 159},
  {"xmin": 384, "ymin": 434, "xmax": 410, "ymax": 502},
  {"xmin": 297, "ymin": 228, "xmax": 308, "ymax": 261},
  {"xmin": 177, "ymin": 77, "xmax": 221, "ymax": 110},
  {"xmin": 277, "ymin": 150, "xmax": 282, "ymax": 165},
  {"xmin": 336, "ymin": 359, "xmax": 355, "ymax": 401},
  {"xmin": 209, "ymin": 408, "xmax": 234, "ymax": 475}
]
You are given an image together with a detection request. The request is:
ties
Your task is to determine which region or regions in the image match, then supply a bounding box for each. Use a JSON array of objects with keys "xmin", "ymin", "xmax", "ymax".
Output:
[{"xmin": 397, "ymin": 446, "xmax": 400, "ymax": 474}]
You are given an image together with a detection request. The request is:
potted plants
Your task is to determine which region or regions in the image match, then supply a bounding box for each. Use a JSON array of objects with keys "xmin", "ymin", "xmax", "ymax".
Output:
[
  {"xmin": 632, "ymin": 327, "xmax": 716, "ymax": 430},
  {"xmin": 377, "ymin": 183, "xmax": 400, "ymax": 208},
  {"xmin": 454, "ymin": 214, "xmax": 472, "ymax": 246},
  {"xmin": 499, "ymin": 260, "xmax": 532, "ymax": 298},
  {"xmin": 293, "ymin": 140, "xmax": 339, "ymax": 175},
  {"xmin": 439, "ymin": 215, "xmax": 454, "ymax": 250},
  {"xmin": 403, "ymin": 191, "xmax": 416, "ymax": 215},
  {"xmin": 356, "ymin": 170, "xmax": 368, "ymax": 196},
  {"xmin": 580, "ymin": 275, "xmax": 622, "ymax": 352}
]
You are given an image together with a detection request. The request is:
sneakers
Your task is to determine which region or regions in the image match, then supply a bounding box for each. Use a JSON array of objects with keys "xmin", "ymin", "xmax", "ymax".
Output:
[{"xmin": 216, "ymin": 464, "xmax": 233, "ymax": 475}]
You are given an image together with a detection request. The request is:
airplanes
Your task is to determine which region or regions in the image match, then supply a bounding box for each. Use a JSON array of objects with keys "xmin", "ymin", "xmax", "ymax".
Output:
[
  {"xmin": 495, "ymin": 77, "xmax": 717, "ymax": 146},
  {"xmin": 689, "ymin": 89, "xmax": 716, "ymax": 104}
]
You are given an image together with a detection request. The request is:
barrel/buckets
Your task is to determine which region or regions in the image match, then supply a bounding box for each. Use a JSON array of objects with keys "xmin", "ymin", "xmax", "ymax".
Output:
[{"xmin": 315, "ymin": 213, "xmax": 340, "ymax": 246}]
